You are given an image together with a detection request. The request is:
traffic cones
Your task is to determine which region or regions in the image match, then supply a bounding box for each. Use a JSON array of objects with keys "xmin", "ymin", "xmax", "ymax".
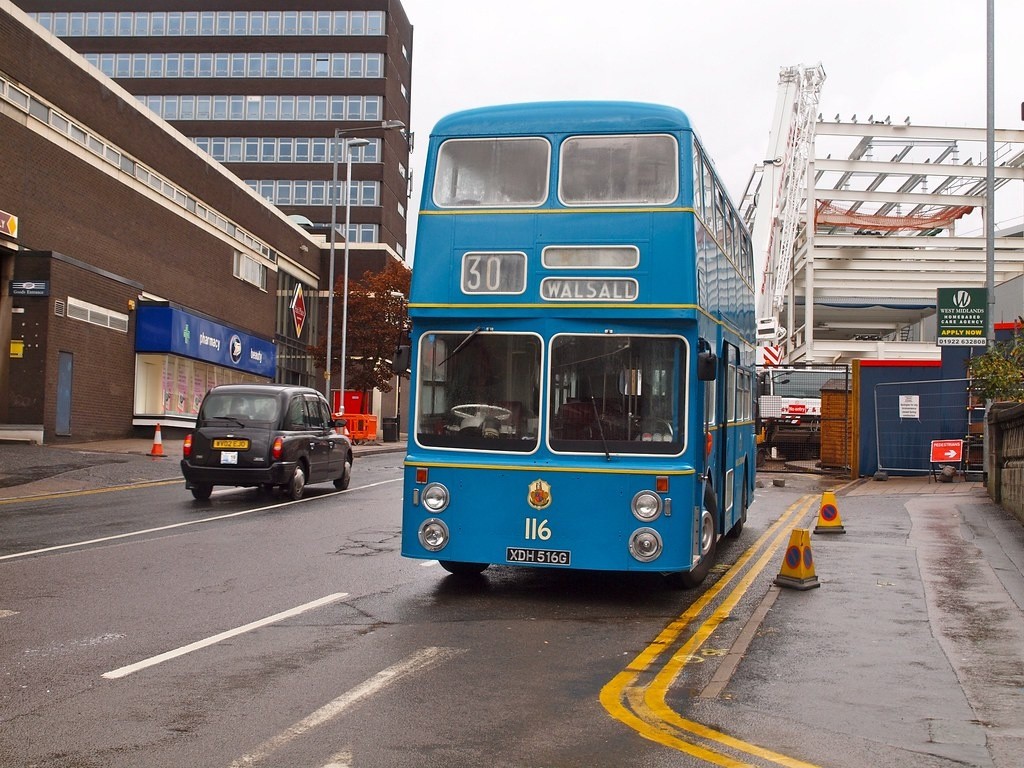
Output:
[
  {"xmin": 812, "ymin": 492, "xmax": 846, "ymax": 535},
  {"xmin": 773, "ymin": 529, "xmax": 821, "ymax": 592},
  {"xmin": 145, "ymin": 422, "xmax": 168, "ymax": 457}
]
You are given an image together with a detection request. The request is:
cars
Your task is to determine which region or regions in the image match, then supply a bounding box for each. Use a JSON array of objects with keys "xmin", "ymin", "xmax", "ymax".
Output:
[{"xmin": 179, "ymin": 382, "xmax": 354, "ymax": 502}]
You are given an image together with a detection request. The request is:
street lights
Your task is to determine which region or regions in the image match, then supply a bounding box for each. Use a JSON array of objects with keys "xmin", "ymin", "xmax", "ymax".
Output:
[
  {"xmin": 336, "ymin": 138, "xmax": 370, "ymax": 417},
  {"xmin": 324, "ymin": 120, "xmax": 406, "ymax": 407}
]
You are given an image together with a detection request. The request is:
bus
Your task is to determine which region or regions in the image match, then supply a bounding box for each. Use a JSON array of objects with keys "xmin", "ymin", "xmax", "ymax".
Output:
[{"xmin": 389, "ymin": 99, "xmax": 759, "ymax": 591}]
